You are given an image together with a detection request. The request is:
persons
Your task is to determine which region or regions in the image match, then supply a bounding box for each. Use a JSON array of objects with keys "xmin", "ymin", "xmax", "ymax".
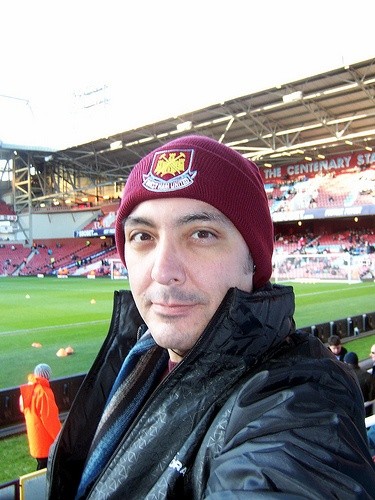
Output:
[
  {"xmin": 269, "ymin": 173, "xmax": 309, "ymax": 199},
  {"xmin": 327, "ymin": 335, "xmax": 348, "ymax": 361},
  {"xmin": 44, "ymin": 132, "xmax": 375, "ymax": 500},
  {"xmin": 359, "ymin": 161, "xmax": 371, "ymax": 170},
  {"xmin": 329, "ymin": 197, "xmax": 334, "ymax": 202},
  {"xmin": 0, "ymin": 240, "xmax": 128, "ymax": 276},
  {"xmin": 271, "ymin": 225, "xmax": 375, "ymax": 277},
  {"xmin": 310, "ymin": 197, "xmax": 316, "ymax": 208},
  {"xmin": 343, "ymin": 352, "xmax": 375, "ymax": 417},
  {"xmin": 20, "ymin": 363, "xmax": 63, "ymax": 470},
  {"xmin": 370, "ymin": 344, "xmax": 375, "ymax": 376}
]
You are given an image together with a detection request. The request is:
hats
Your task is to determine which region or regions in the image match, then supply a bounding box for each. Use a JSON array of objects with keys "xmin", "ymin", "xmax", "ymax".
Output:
[
  {"xmin": 34, "ymin": 363, "xmax": 51, "ymax": 380},
  {"xmin": 114, "ymin": 134, "xmax": 274, "ymax": 290},
  {"xmin": 343, "ymin": 352, "xmax": 359, "ymax": 365}
]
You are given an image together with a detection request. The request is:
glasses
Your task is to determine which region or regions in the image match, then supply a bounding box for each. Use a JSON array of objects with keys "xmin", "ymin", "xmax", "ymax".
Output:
[{"xmin": 370, "ymin": 351, "xmax": 375, "ymax": 354}]
